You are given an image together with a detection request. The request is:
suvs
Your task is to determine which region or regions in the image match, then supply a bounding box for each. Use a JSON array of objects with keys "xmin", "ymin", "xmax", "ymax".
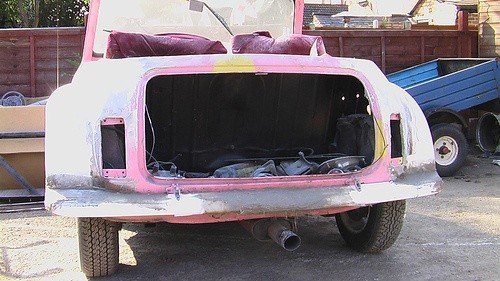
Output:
[{"xmin": 45, "ymin": 1, "xmax": 442, "ymax": 279}]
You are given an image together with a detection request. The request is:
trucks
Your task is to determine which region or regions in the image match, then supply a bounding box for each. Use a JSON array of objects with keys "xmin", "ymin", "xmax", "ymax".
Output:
[{"xmin": 386, "ymin": 54, "xmax": 499, "ymax": 181}]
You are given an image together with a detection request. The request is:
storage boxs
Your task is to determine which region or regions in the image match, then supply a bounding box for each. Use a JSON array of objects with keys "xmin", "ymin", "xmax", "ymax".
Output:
[
  {"xmin": 0, "ymin": 94, "xmax": 51, "ymax": 188},
  {"xmin": 384, "ymin": 57, "xmax": 500, "ymax": 114}
]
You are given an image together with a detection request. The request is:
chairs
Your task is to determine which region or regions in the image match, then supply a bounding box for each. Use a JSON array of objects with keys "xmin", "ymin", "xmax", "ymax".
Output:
[
  {"xmin": 105, "ymin": 30, "xmax": 228, "ymax": 58},
  {"xmin": 231, "ymin": 30, "xmax": 327, "ymax": 56}
]
